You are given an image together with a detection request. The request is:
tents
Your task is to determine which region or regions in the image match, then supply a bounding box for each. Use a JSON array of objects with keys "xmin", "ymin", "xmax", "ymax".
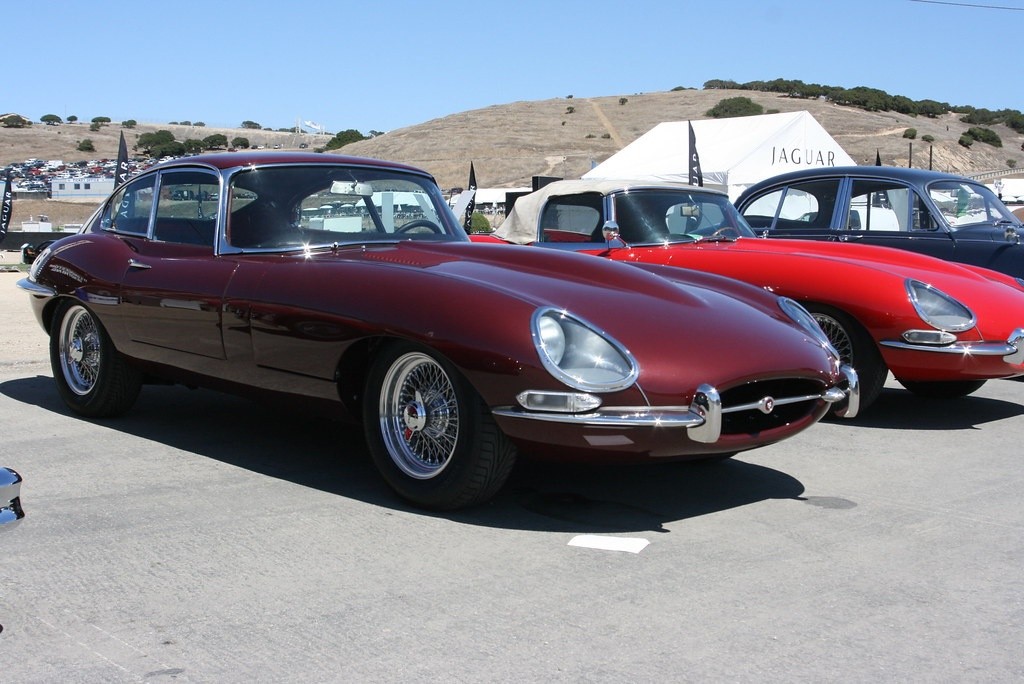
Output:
[{"xmin": 579, "ymin": 109, "xmax": 898, "ymax": 230}]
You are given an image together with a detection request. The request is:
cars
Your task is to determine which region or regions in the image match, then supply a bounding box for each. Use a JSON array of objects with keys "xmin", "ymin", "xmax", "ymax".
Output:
[
  {"xmin": 461, "ymin": 177, "xmax": 1024, "ymax": 422},
  {"xmin": 690, "ymin": 166, "xmax": 1024, "ymax": 283},
  {"xmin": 15, "ymin": 150, "xmax": 860, "ymax": 513}
]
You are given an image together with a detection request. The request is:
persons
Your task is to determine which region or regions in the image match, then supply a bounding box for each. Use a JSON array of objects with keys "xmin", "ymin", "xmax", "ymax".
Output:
[{"xmin": 995, "ymin": 182, "xmax": 1004, "ymax": 200}]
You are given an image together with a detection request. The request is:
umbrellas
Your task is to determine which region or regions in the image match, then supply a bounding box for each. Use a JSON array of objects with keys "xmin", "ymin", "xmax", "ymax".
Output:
[
  {"xmin": 341, "ymin": 204, "xmax": 354, "ymax": 208},
  {"xmin": 319, "ymin": 205, "xmax": 333, "ymax": 209},
  {"xmin": 397, "ymin": 204, "xmax": 402, "ymax": 213}
]
